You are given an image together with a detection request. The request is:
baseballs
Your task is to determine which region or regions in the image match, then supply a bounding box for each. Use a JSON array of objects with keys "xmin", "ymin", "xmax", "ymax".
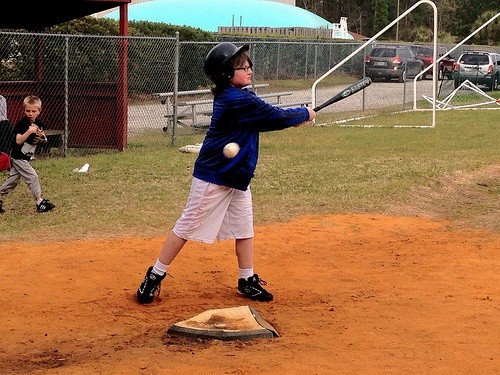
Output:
[{"xmin": 224, "ymin": 143, "xmax": 240, "ymax": 158}]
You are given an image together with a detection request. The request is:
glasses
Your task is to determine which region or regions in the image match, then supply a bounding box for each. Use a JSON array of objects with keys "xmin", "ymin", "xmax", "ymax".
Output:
[{"xmin": 233, "ymin": 65, "xmax": 252, "ymax": 71}]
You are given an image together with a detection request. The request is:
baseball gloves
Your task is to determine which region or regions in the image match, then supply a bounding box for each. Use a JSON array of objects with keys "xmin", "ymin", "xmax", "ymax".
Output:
[{"xmin": 31, "ymin": 123, "xmax": 47, "ymax": 144}]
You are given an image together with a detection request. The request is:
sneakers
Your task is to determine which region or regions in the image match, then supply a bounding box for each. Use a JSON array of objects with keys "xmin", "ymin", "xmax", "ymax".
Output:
[
  {"xmin": 137, "ymin": 265, "xmax": 166, "ymax": 303},
  {"xmin": 238, "ymin": 274, "xmax": 273, "ymax": 301},
  {"xmin": 37, "ymin": 198, "xmax": 56, "ymax": 211},
  {"xmin": 0, "ymin": 200, "xmax": 6, "ymax": 212}
]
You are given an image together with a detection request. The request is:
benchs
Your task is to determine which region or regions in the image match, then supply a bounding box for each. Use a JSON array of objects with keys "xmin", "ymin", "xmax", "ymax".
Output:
[{"xmin": 153, "ymin": 83, "xmax": 314, "ymax": 132}]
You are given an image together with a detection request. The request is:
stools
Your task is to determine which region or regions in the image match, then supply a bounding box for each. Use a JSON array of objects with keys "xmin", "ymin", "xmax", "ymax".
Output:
[{"xmin": 33, "ymin": 130, "xmax": 66, "ymax": 161}]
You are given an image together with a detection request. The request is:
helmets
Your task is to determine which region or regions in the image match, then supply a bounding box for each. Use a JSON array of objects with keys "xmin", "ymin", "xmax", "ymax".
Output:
[{"xmin": 204, "ymin": 42, "xmax": 249, "ymax": 78}]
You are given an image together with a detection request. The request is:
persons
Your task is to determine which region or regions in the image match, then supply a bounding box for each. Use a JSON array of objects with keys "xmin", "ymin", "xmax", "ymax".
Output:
[
  {"xmin": 0, "ymin": 95, "xmax": 56, "ymax": 212},
  {"xmin": 136, "ymin": 42, "xmax": 316, "ymax": 304}
]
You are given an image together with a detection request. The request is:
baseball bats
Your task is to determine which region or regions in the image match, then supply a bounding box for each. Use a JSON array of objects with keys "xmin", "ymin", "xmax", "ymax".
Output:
[{"xmin": 313, "ymin": 76, "xmax": 371, "ymax": 113}]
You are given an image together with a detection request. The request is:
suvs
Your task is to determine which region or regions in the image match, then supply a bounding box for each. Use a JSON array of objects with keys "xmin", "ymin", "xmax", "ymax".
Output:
[
  {"xmin": 451, "ymin": 50, "xmax": 500, "ymax": 92},
  {"xmin": 366, "ymin": 45, "xmax": 426, "ymax": 83},
  {"xmin": 410, "ymin": 44, "xmax": 456, "ymax": 80}
]
